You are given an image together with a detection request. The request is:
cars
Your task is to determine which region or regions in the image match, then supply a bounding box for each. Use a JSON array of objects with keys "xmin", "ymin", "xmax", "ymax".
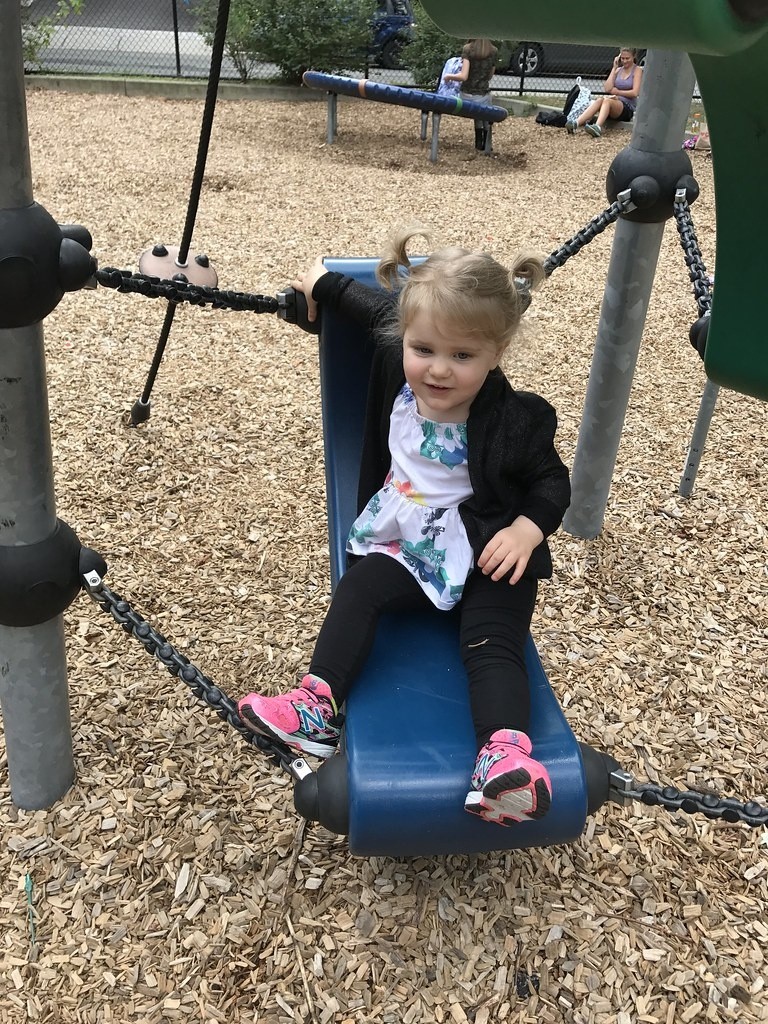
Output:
[{"xmin": 511, "ymin": 41, "xmax": 647, "ymax": 77}]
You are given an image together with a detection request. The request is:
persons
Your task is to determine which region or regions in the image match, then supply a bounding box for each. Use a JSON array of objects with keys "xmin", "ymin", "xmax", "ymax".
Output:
[
  {"xmin": 239, "ymin": 231, "xmax": 572, "ymax": 828},
  {"xmin": 565, "ymin": 47, "xmax": 642, "ymax": 138},
  {"xmin": 444, "ymin": 38, "xmax": 498, "ymax": 151}
]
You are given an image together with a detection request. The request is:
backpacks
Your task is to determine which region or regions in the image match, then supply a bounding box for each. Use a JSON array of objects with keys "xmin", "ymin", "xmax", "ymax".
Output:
[
  {"xmin": 567, "ymin": 77, "xmax": 592, "ymax": 121},
  {"xmin": 438, "ymin": 56, "xmax": 464, "ymax": 97}
]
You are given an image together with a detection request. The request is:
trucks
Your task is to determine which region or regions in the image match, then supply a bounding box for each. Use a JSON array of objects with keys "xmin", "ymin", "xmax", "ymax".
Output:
[{"xmin": 368, "ymin": 0, "xmax": 415, "ymax": 69}]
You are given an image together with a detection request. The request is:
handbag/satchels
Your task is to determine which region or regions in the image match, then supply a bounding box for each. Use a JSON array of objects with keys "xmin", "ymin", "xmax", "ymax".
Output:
[{"xmin": 695, "ymin": 119, "xmax": 711, "ymax": 151}]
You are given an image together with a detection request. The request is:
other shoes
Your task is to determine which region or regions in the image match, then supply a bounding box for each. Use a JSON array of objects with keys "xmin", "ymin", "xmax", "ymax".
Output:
[
  {"xmin": 585, "ymin": 123, "xmax": 601, "ymax": 138},
  {"xmin": 565, "ymin": 120, "xmax": 578, "ymax": 134}
]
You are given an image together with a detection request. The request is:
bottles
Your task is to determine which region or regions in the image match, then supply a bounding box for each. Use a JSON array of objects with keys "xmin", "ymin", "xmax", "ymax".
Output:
[{"xmin": 692, "ymin": 114, "xmax": 701, "ymax": 133}]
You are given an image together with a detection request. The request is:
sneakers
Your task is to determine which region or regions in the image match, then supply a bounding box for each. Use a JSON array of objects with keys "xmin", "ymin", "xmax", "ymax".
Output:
[
  {"xmin": 237, "ymin": 674, "xmax": 345, "ymax": 759},
  {"xmin": 463, "ymin": 729, "xmax": 553, "ymax": 828}
]
